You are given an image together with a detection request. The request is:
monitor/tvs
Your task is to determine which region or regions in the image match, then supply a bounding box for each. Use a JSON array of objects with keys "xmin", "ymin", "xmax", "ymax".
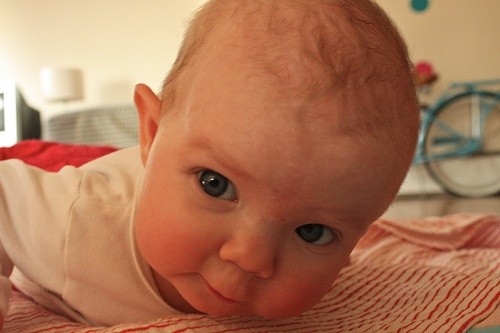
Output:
[{"xmin": 0, "ymin": 82, "xmax": 41, "ymax": 148}]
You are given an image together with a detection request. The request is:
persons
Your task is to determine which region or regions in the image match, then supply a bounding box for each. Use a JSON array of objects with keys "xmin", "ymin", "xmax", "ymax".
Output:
[{"xmin": 0, "ymin": 0, "xmax": 423, "ymax": 333}]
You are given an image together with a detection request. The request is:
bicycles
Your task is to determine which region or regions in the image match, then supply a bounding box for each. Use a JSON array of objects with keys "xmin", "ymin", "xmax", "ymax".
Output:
[{"xmin": 411, "ymin": 63, "xmax": 500, "ymax": 198}]
